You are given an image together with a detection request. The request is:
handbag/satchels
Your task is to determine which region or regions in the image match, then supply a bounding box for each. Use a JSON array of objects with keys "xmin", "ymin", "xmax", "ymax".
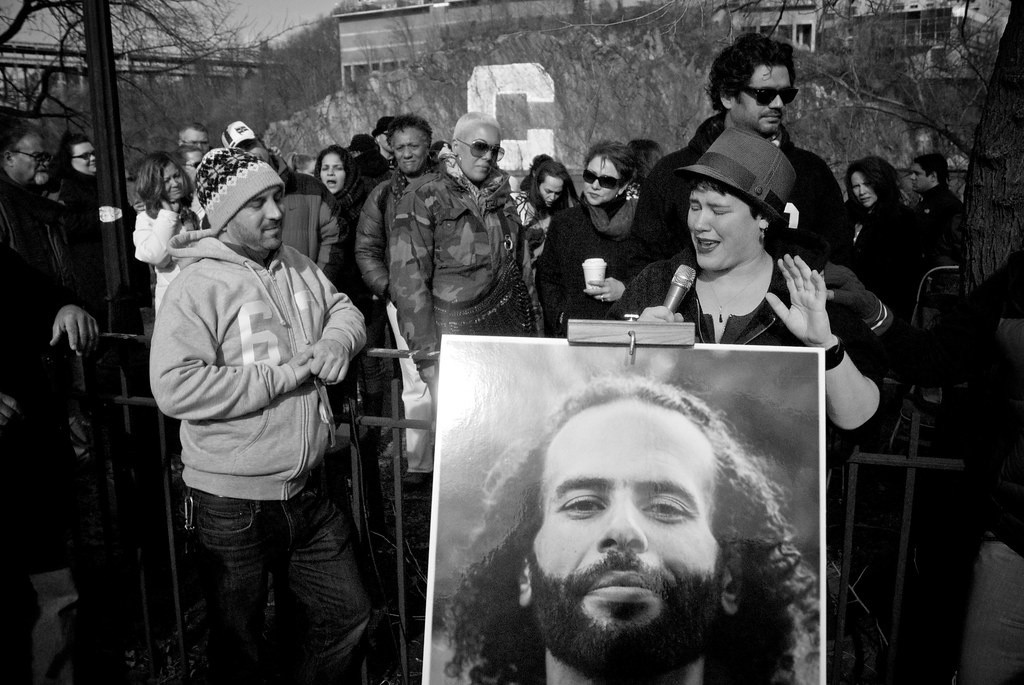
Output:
[{"xmin": 431, "ymin": 254, "xmax": 538, "ymax": 341}]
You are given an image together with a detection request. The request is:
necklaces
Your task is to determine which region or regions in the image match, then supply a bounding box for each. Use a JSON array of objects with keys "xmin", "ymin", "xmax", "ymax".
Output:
[{"xmin": 707, "ymin": 258, "xmax": 765, "ymax": 323}]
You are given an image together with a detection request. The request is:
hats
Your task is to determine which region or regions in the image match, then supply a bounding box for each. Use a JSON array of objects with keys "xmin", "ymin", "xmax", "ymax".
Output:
[
  {"xmin": 673, "ymin": 127, "xmax": 797, "ymax": 224},
  {"xmin": 194, "ymin": 147, "xmax": 285, "ymax": 237},
  {"xmin": 372, "ymin": 116, "xmax": 395, "ymax": 137},
  {"xmin": 221, "ymin": 121, "xmax": 256, "ymax": 148}
]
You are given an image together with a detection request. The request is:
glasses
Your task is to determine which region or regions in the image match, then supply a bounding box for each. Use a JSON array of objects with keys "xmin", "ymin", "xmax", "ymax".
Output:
[
  {"xmin": 71, "ymin": 150, "xmax": 96, "ymax": 160},
  {"xmin": 10, "ymin": 150, "xmax": 52, "ymax": 162},
  {"xmin": 742, "ymin": 85, "xmax": 798, "ymax": 105},
  {"xmin": 180, "ymin": 161, "xmax": 201, "ymax": 169},
  {"xmin": 455, "ymin": 137, "xmax": 505, "ymax": 162},
  {"xmin": 582, "ymin": 169, "xmax": 625, "ymax": 189}
]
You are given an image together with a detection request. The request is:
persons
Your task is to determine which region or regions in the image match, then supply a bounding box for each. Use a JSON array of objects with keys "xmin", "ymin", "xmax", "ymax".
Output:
[
  {"xmin": 222, "ymin": 115, "xmax": 535, "ymax": 601},
  {"xmin": 604, "ymin": 126, "xmax": 881, "ymax": 430},
  {"xmin": 446, "ymin": 378, "xmax": 820, "ymax": 685},
  {"xmin": 624, "ymin": 35, "xmax": 1024, "ymax": 685},
  {"xmin": 512, "ymin": 138, "xmax": 662, "ymax": 338},
  {"xmin": 0, "ymin": 119, "xmax": 208, "ymax": 685},
  {"xmin": 150, "ymin": 146, "xmax": 373, "ymax": 685}
]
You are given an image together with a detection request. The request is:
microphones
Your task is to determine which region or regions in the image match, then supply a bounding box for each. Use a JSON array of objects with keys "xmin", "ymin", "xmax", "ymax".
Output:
[{"xmin": 664, "ymin": 264, "xmax": 695, "ymax": 313}]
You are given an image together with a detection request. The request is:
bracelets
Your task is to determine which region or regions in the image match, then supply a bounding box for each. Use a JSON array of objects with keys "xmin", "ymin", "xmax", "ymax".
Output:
[{"xmin": 826, "ymin": 336, "xmax": 845, "ymax": 371}]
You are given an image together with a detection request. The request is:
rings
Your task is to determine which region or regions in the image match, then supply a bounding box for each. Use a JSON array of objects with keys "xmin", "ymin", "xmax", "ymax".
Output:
[{"xmin": 601, "ymin": 294, "xmax": 604, "ymax": 302}]
